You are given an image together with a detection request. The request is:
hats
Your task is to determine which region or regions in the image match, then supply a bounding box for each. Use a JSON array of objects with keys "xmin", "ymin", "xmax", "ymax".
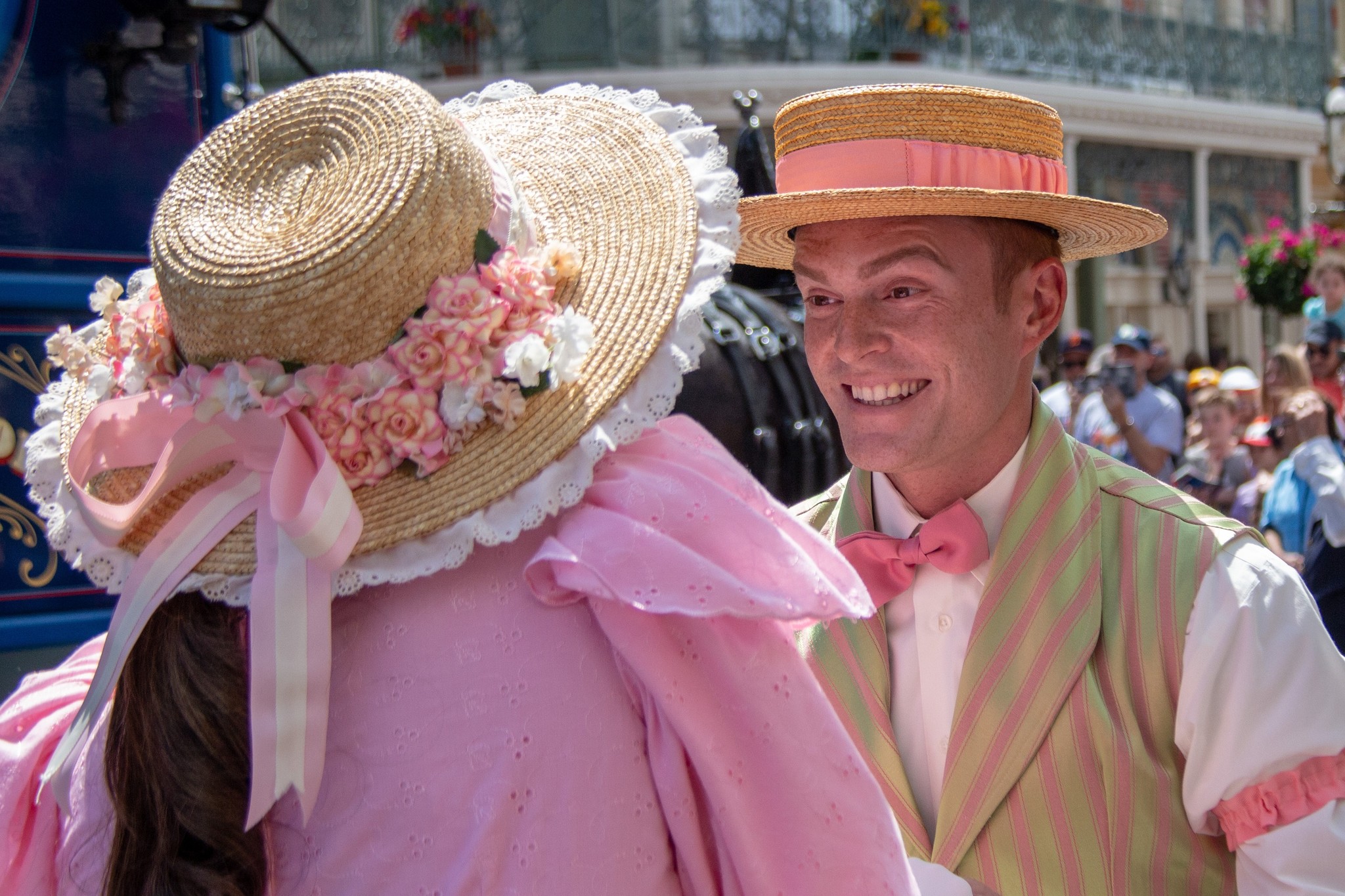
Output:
[
  {"xmin": 733, "ymin": 83, "xmax": 1168, "ymax": 271},
  {"xmin": 24, "ymin": 69, "xmax": 744, "ymax": 835},
  {"xmin": 1218, "ymin": 365, "xmax": 1261, "ymax": 392},
  {"xmin": 1150, "ymin": 340, "xmax": 1171, "ymax": 357},
  {"xmin": 1187, "ymin": 366, "xmax": 1222, "ymax": 391},
  {"xmin": 1303, "ymin": 321, "xmax": 1342, "ymax": 345},
  {"xmin": 1111, "ymin": 322, "xmax": 1152, "ymax": 352},
  {"xmin": 1058, "ymin": 329, "xmax": 1093, "ymax": 352},
  {"xmin": 1237, "ymin": 415, "xmax": 1274, "ymax": 447}
]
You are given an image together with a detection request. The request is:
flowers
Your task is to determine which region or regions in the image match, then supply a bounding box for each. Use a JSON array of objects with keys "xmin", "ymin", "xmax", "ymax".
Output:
[
  {"xmin": 1235, "ymin": 217, "xmax": 1345, "ymax": 319},
  {"xmin": 46, "ymin": 241, "xmax": 595, "ymax": 488}
]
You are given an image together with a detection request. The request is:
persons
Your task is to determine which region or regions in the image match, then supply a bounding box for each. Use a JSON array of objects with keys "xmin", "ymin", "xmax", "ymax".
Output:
[
  {"xmin": 1031, "ymin": 241, "xmax": 1345, "ymax": 651},
  {"xmin": 0, "ymin": 68, "xmax": 974, "ymax": 896},
  {"xmin": 736, "ymin": 84, "xmax": 1345, "ymax": 896}
]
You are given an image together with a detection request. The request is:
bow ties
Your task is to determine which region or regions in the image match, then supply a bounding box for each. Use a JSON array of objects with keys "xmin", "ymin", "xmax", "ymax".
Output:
[{"xmin": 837, "ymin": 498, "xmax": 991, "ymax": 608}]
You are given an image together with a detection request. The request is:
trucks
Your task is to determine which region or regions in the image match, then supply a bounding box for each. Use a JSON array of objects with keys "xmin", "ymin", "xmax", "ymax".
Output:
[{"xmin": 0, "ymin": 0, "xmax": 276, "ymax": 651}]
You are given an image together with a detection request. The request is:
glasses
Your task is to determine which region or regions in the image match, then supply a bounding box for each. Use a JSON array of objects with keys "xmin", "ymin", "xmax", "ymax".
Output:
[
  {"xmin": 1305, "ymin": 347, "xmax": 1331, "ymax": 360},
  {"xmin": 1063, "ymin": 360, "xmax": 1087, "ymax": 368}
]
